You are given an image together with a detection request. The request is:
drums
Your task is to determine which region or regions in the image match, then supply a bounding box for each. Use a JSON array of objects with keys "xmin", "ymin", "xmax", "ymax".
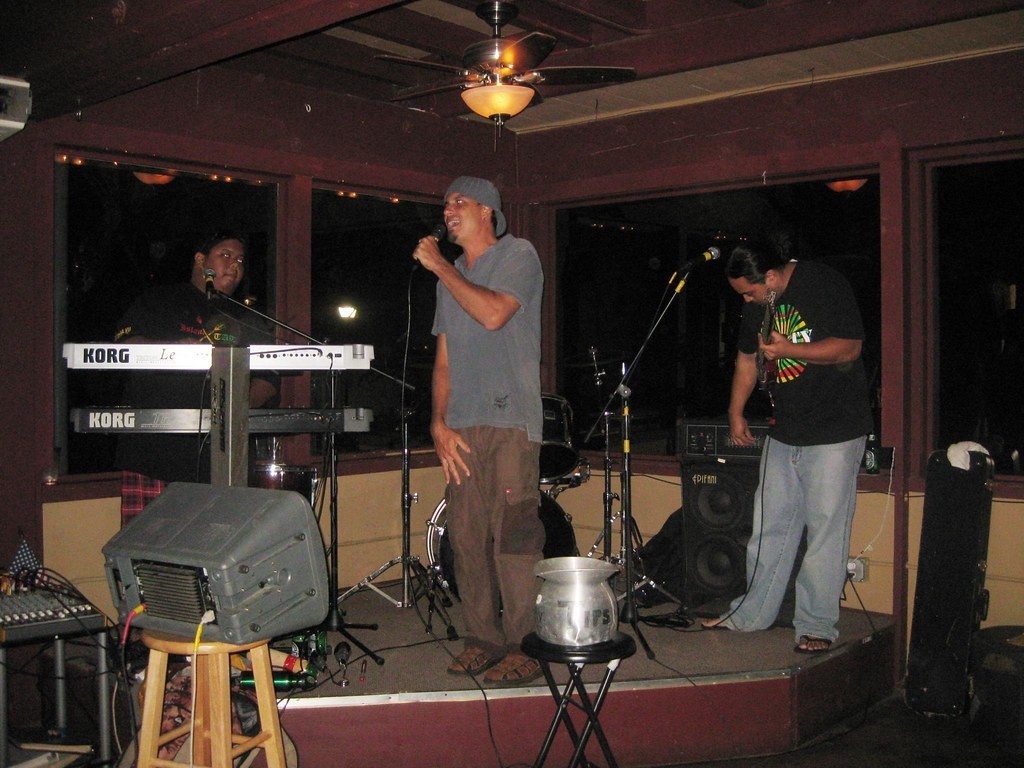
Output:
[
  {"xmin": 425, "ymin": 489, "xmax": 578, "ymax": 617},
  {"xmin": 539, "ymin": 390, "xmax": 580, "ymax": 484},
  {"xmin": 248, "ymin": 464, "xmax": 318, "ymax": 509},
  {"xmin": 550, "ymin": 457, "xmax": 591, "ymax": 485}
]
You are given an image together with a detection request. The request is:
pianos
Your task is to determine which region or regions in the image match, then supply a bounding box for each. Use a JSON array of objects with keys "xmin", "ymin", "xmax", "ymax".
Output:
[{"xmin": 61, "ymin": 340, "xmax": 387, "ymax": 667}]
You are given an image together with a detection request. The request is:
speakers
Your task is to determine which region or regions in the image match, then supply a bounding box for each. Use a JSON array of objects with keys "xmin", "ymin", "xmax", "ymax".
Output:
[
  {"xmin": 101, "ymin": 483, "xmax": 330, "ymax": 644},
  {"xmin": 680, "ymin": 411, "xmax": 806, "ymax": 628}
]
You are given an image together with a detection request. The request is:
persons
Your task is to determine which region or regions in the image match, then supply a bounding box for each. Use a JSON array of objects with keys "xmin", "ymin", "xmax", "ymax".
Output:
[
  {"xmin": 698, "ymin": 234, "xmax": 873, "ymax": 653},
  {"xmin": 115, "ymin": 230, "xmax": 281, "ymax": 486},
  {"xmin": 412, "ymin": 175, "xmax": 543, "ymax": 682}
]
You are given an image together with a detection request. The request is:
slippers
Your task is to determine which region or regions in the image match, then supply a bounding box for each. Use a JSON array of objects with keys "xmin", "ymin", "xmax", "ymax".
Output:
[
  {"xmin": 793, "ymin": 630, "xmax": 830, "ymax": 656},
  {"xmin": 702, "ymin": 617, "xmax": 728, "ymax": 629}
]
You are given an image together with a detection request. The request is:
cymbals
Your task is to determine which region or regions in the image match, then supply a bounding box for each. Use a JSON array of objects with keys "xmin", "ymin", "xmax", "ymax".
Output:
[
  {"xmin": 591, "ymin": 408, "xmax": 660, "ymax": 420},
  {"xmin": 563, "ymin": 348, "xmax": 626, "ymax": 367}
]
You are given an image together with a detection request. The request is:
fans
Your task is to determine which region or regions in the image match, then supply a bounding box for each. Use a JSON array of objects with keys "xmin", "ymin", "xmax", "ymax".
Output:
[{"xmin": 372, "ymin": 1, "xmax": 636, "ymax": 120}]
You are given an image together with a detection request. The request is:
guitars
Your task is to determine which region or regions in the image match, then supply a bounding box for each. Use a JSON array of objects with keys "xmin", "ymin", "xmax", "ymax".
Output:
[{"xmin": 754, "ymin": 289, "xmax": 778, "ymax": 392}]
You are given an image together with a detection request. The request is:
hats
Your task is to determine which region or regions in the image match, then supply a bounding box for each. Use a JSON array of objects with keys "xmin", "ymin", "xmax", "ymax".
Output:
[{"xmin": 444, "ymin": 175, "xmax": 506, "ymax": 237}]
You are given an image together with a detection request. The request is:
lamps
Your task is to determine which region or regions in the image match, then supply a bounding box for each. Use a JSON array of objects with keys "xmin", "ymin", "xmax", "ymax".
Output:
[{"xmin": 461, "ymin": 73, "xmax": 535, "ymax": 150}]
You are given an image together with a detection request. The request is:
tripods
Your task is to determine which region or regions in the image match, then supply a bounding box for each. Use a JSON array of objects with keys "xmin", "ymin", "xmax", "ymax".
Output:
[
  {"xmin": 217, "ymin": 292, "xmax": 460, "ymax": 667},
  {"xmin": 586, "ymin": 365, "xmax": 693, "ymax": 661}
]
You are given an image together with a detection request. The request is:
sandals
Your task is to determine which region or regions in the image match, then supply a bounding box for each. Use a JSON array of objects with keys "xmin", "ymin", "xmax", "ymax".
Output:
[
  {"xmin": 449, "ymin": 645, "xmax": 497, "ymax": 675},
  {"xmin": 483, "ymin": 646, "xmax": 544, "ymax": 686}
]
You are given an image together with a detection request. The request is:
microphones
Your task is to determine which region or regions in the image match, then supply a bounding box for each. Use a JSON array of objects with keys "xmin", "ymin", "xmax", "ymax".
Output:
[
  {"xmin": 205, "ymin": 268, "xmax": 217, "ymax": 300},
  {"xmin": 675, "ymin": 246, "xmax": 721, "ymax": 272},
  {"xmin": 413, "ymin": 223, "xmax": 447, "ymax": 272}
]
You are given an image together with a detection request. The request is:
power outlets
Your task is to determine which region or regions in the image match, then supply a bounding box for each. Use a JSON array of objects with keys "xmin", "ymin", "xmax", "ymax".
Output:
[{"xmin": 847, "ymin": 558, "xmax": 868, "ymax": 583}]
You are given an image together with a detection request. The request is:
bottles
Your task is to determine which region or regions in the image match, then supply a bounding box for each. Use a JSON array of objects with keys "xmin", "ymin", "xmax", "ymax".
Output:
[
  {"xmin": 866, "ymin": 429, "xmax": 879, "ymax": 474},
  {"xmin": 238, "ymin": 629, "xmax": 327, "ymax": 692}
]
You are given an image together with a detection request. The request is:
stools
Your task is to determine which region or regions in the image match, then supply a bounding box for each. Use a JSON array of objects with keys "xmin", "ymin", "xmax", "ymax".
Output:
[
  {"xmin": 521, "ymin": 631, "xmax": 637, "ymax": 768},
  {"xmin": 136, "ymin": 628, "xmax": 286, "ymax": 768}
]
